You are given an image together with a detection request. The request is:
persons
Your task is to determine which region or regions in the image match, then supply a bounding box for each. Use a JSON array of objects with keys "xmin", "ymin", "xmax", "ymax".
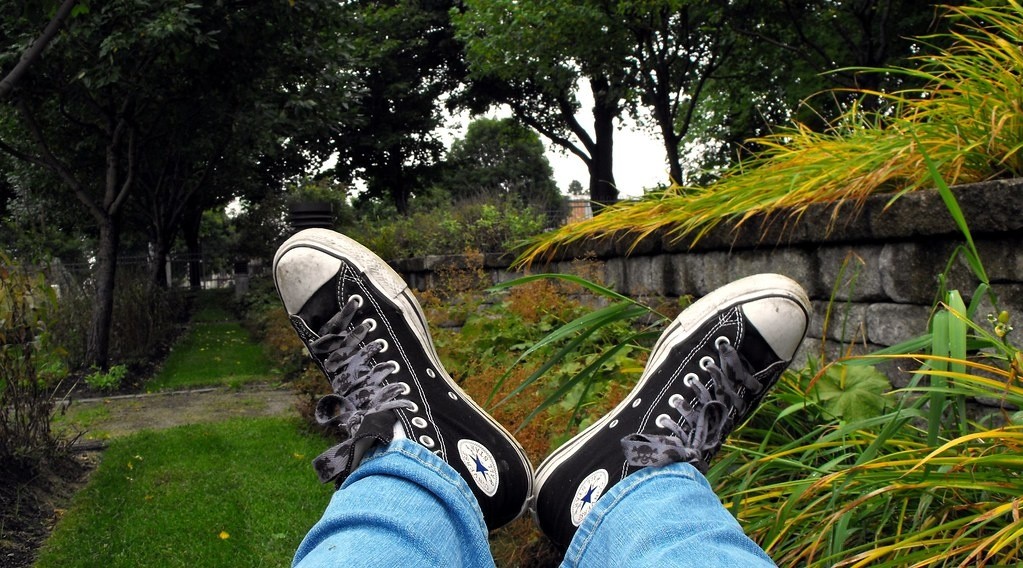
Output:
[{"xmin": 272, "ymin": 228, "xmax": 813, "ymax": 568}]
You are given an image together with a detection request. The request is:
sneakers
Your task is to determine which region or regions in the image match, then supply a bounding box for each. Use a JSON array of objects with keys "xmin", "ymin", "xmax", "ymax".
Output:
[
  {"xmin": 531, "ymin": 273, "xmax": 812, "ymax": 552},
  {"xmin": 272, "ymin": 227, "xmax": 535, "ymax": 530}
]
